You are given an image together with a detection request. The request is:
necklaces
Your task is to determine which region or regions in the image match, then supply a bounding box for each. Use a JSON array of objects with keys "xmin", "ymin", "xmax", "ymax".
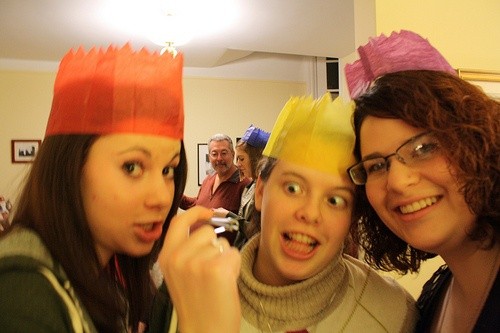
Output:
[
  {"xmin": 258, "ymin": 294, "xmax": 337, "ymax": 333},
  {"xmin": 440, "ymin": 250, "xmax": 499, "ymax": 333}
]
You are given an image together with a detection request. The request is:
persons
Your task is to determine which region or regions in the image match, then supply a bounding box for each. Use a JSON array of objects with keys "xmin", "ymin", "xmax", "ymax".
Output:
[
  {"xmin": 0, "ymin": 43, "xmax": 187, "ymax": 333},
  {"xmin": 182, "ymin": 125, "xmax": 270, "ymax": 248},
  {"xmin": 344, "ymin": 30, "xmax": 500, "ymax": 333},
  {"xmin": 158, "ymin": 88, "xmax": 418, "ymax": 333}
]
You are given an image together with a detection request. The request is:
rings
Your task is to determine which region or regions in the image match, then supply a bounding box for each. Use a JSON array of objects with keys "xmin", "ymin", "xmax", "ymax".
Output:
[{"xmin": 211, "ymin": 240, "xmax": 223, "ymax": 251}]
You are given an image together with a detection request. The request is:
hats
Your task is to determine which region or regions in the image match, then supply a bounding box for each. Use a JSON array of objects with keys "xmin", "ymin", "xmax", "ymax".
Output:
[
  {"xmin": 344, "ymin": 30, "xmax": 467, "ymax": 101},
  {"xmin": 260, "ymin": 93, "xmax": 357, "ymax": 176},
  {"xmin": 240, "ymin": 125, "xmax": 273, "ymax": 145},
  {"xmin": 44, "ymin": 43, "xmax": 187, "ymax": 140}
]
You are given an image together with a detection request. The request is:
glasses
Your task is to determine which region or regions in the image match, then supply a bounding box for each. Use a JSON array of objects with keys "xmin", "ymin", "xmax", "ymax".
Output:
[{"xmin": 346, "ymin": 129, "xmax": 443, "ymax": 185}]
